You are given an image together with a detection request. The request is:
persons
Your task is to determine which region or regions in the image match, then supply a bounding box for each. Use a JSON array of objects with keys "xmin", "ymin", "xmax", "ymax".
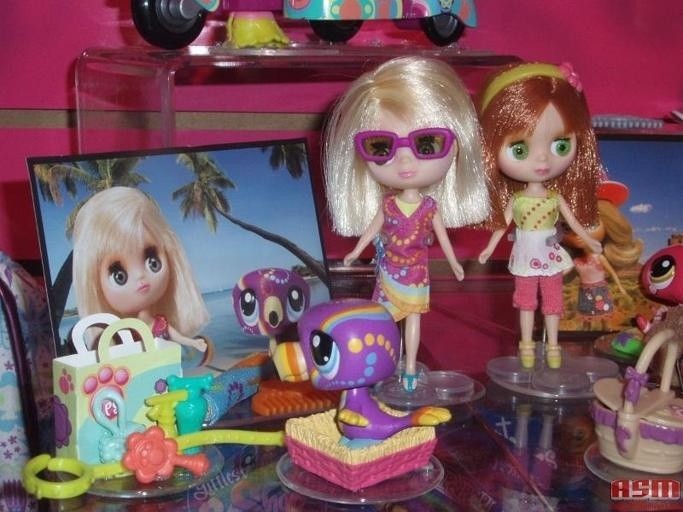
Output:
[
  {"xmin": 465, "ymin": 59, "xmax": 603, "ymax": 368},
  {"xmin": 557, "ymin": 157, "xmax": 642, "ymax": 313},
  {"xmin": 321, "ymin": 55, "xmax": 494, "ymax": 391},
  {"xmin": 68, "ymin": 183, "xmax": 208, "ymax": 353}
]
